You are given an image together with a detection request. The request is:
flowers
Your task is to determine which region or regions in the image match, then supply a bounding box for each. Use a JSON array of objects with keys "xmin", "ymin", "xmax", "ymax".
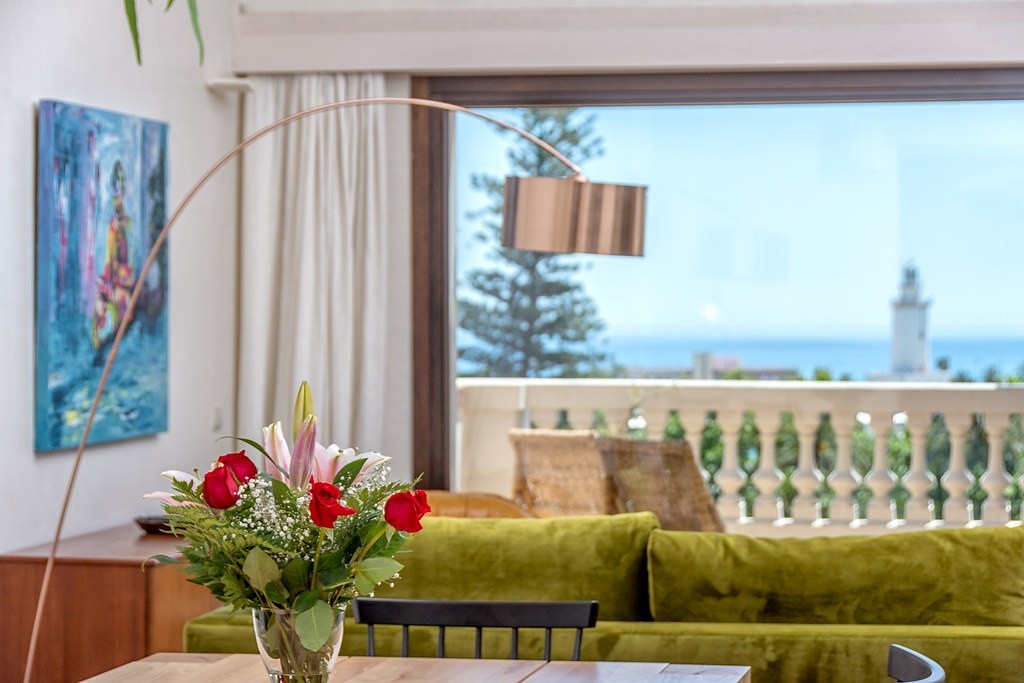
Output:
[{"xmin": 140, "ymin": 380, "xmax": 433, "ymax": 653}]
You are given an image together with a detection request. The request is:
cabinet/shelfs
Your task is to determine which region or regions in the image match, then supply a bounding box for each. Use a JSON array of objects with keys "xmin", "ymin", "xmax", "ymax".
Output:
[{"xmin": 0, "ymin": 520, "xmax": 224, "ymax": 683}]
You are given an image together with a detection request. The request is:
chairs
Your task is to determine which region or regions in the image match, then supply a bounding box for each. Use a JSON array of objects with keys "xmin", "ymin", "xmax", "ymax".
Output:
[
  {"xmin": 352, "ymin": 598, "xmax": 599, "ymax": 662},
  {"xmin": 887, "ymin": 643, "xmax": 946, "ymax": 683}
]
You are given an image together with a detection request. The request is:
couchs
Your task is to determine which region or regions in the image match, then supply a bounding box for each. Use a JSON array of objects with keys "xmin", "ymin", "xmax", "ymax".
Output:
[{"xmin": 179, "ymin": 601, "xmax": 1024, "ymax": 680}]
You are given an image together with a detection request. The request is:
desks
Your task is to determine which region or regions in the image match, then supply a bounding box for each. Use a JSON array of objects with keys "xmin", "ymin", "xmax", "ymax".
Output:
[{"xmin": 76, "ymin": 651, "xmax": 752, "ymax": 683}]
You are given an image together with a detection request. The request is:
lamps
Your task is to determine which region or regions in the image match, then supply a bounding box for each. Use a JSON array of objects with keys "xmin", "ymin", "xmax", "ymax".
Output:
[{"xmin": 20, "ymin": 96, "xmax": 648, "ymax": 683}]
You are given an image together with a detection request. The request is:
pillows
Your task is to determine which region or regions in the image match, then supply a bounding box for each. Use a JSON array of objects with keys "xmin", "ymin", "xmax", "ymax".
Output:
[
  {"xmin": 350, "ymin": 511, "xmax": 658, "ymax": 621},
  {"xmin": 644, "ymin": 523, "xmax": 1022, "ymax": 626}
]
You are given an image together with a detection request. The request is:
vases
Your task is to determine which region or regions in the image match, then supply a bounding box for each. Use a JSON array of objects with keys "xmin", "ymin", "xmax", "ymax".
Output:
[{"xmin": 251, "ymin": 602, "xmax": 348, "ymax": 683}]
